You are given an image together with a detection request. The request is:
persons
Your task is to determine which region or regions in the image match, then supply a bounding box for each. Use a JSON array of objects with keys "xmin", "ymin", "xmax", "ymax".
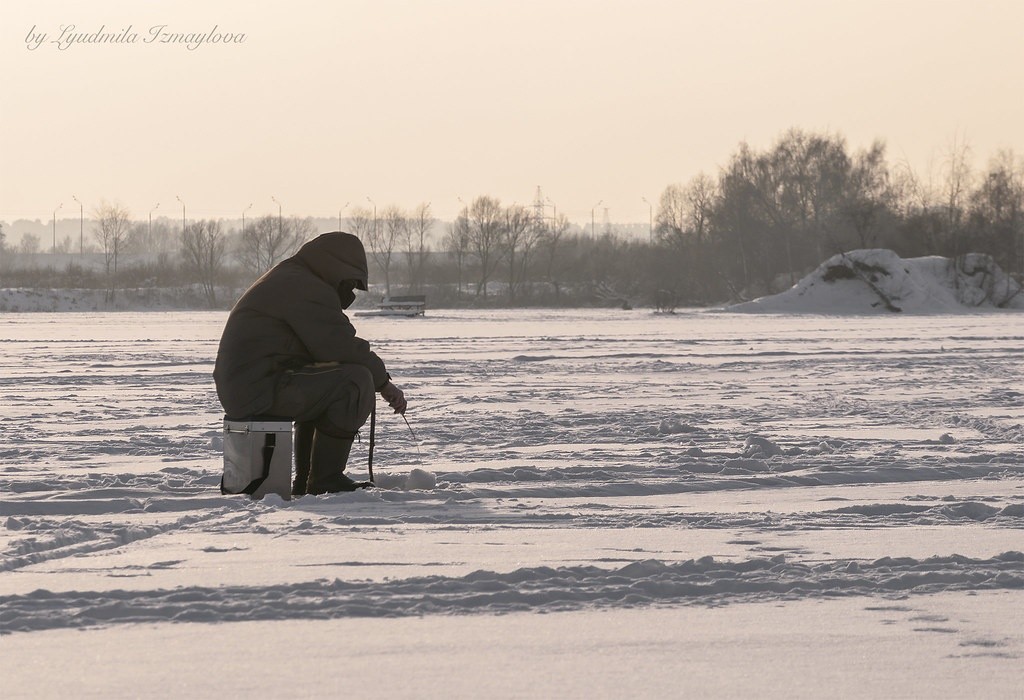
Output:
[{"xmin": 212, "ymin": 230, "xmax": 408, "ymax": 496}]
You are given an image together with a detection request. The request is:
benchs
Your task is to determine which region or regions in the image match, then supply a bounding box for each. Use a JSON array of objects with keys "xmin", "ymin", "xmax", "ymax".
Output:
[{"xmin": 382, "ymin": 296, "xmax": 425, "ymax": 316}]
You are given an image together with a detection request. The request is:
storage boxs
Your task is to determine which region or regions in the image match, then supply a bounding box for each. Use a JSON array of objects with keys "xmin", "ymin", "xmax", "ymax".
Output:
[{"xmin": 220, "ymin": 420, "xmax": 292, "ymax": 501}]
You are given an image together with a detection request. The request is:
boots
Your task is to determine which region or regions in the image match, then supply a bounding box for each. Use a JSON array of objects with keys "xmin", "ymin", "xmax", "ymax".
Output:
[{"xmin": 292, "ymin": 421, "xmax": 375, "ymax": 496}]
